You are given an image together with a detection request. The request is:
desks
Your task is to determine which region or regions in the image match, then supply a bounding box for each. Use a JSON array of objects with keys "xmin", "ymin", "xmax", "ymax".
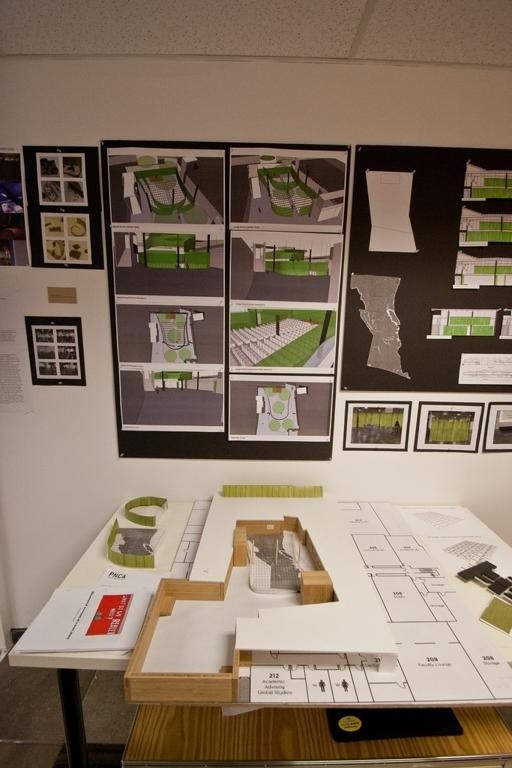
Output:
[{"xmin": 8, "ymin": 501, "xmax": 512, "ymax": 767}]
